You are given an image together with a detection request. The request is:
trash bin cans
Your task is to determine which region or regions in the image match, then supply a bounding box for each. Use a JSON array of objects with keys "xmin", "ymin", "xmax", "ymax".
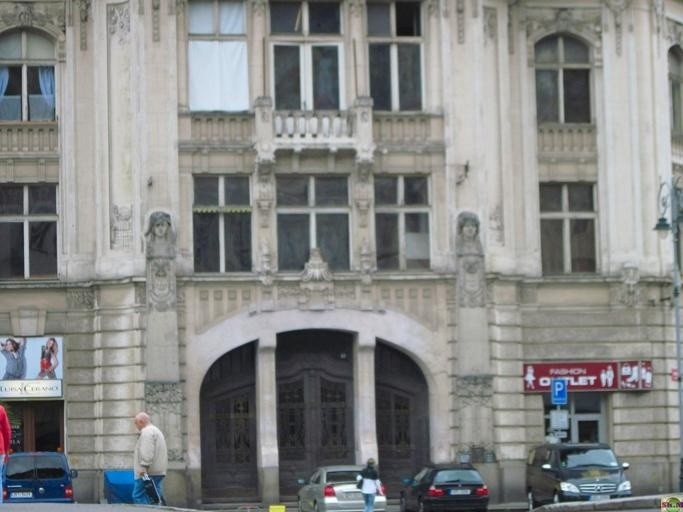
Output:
[{"xmin": 103, "ymin": 469, "xmax": 135, "ymax": 504}]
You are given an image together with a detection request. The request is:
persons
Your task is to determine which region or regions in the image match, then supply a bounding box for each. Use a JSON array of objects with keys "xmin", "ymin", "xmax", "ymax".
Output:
[
  {"xmin": 0, "ymin": 338, "xmax": 27, "ymax": 380},
  {"xmin": 33, "ymin": 338, "xmax": 58, "ymax": 379},
  {"xmin": 144, "ymin": 214, "xmax": 175, "ymax": 256},
  {"xmin": 355, "ymin": 457, "xmax": 383, "ymax": 512},
  {"xmin": 131, "ymin": 412, "xmax": 167, "ymax": 505},
  {"xmin": 0, "ymin": 404, "xmax": 11, "ymax": 504},
  {"xmin": 456, "ymin": 211, "xmax": 484, "ymax": 256}
]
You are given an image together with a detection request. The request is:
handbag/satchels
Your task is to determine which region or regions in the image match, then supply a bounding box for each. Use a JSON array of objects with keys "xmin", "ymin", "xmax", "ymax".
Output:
[{"xmin": 357, "ymin": 480, "xmax": 363, "ymax": 489}]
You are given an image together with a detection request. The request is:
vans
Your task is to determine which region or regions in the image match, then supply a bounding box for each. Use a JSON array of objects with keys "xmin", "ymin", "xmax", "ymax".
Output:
[
  {"xmin": 0, "ymin": 449, "xmax": 77, "ymax": 507},
  {"xmin": 524, "ymin": 438, "xmax": 631, "ymax": 511}
]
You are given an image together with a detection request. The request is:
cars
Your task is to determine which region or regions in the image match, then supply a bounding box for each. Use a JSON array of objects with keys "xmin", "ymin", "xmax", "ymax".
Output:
[
  {"xmin": 398, "ymin": 462, "xmax": 491, "ymax": 512},
  {"xmin": 293, "ymin": 461, "xmax": 388, "ymax": 512}
]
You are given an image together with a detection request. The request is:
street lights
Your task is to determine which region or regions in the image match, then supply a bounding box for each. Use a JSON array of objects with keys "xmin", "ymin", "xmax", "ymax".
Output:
[{"xmin": 651, "ymin": 173, "xmax": 682, "ymax": 493}]
